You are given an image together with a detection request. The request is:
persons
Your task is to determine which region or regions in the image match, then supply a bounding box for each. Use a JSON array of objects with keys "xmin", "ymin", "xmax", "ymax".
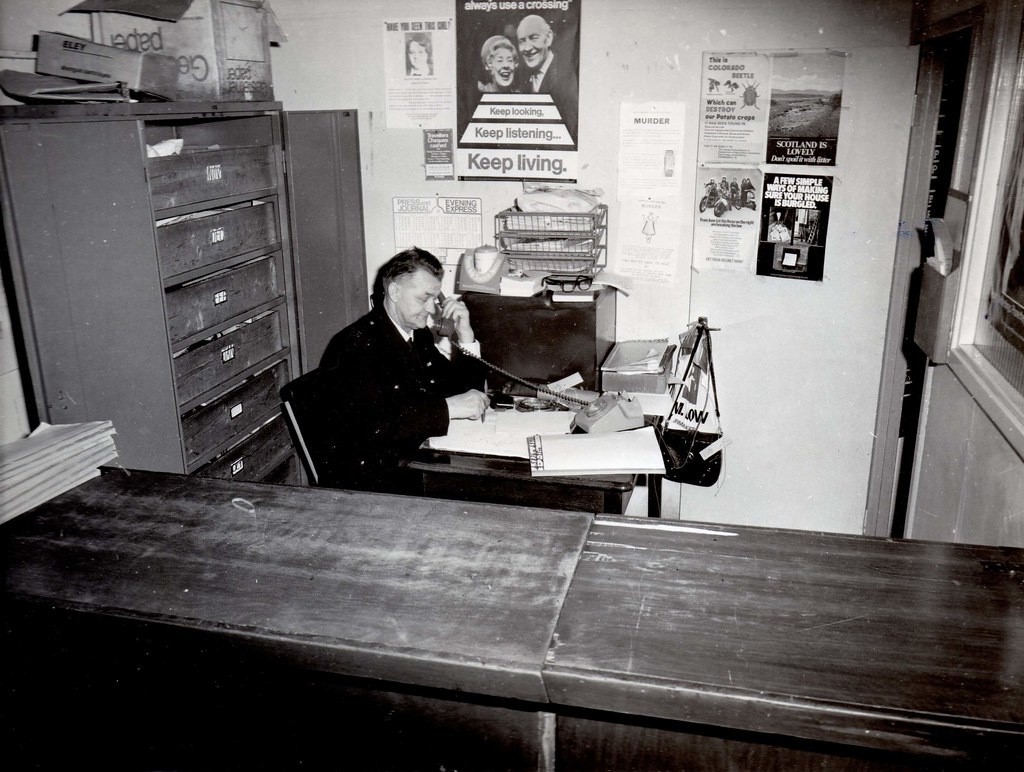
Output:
[
  {"xmin": 477, "ymin": 35, "xmax": 519, "ymax": 93},
  {"xmin": 699, "ymin": 175, "xmax": 756, "ymax": 211},
  {"xmin": 406, "ymin": 34, "xmax": 433, "ymax": 76},
  {"xmin": 516, "ymin": 14, "xmax": 578, "ymax": 137},
  {"xmin": 316, "ymin": 245, "xmax": 491, "ymax": 474}
]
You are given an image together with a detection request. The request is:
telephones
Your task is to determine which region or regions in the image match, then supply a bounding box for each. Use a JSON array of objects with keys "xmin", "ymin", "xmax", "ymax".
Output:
[{"xmin": 435, "ymin": 291, "xmax": 455, "ymax": 337}]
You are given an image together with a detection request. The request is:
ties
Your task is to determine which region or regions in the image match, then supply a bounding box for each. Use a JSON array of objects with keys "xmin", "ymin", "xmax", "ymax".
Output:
[{"xmin": 530, "ymin": 74, "xmax": 538, "ymax": 94}]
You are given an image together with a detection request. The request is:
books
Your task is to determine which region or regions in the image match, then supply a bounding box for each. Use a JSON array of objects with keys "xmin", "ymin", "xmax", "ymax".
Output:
[
  {"xmin": 527, "ymin": 426, "xmax": 667, "ymax": 477},
  {"xmin": 0, "ymin": 420, "xmax": 119, "ymax": 526},
  {"xmin": 601, "ymin": 337, "xmax": 677, "ymax": 398},
  {"xmin": 552, "ymin": 290, "xmax": 595, "ymax": 302}
]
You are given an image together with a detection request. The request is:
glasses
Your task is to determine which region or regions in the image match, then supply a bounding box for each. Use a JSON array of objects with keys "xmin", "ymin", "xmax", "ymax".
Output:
[{"xmin": 541, "ymin": 275, "xmax": 593, "ymax": 293}]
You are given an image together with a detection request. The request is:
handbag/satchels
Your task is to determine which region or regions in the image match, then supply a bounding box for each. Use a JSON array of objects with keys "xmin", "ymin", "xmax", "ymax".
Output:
[{"xmin": 660, "ymin": 427, "xmax": 722, "ymax": 487}]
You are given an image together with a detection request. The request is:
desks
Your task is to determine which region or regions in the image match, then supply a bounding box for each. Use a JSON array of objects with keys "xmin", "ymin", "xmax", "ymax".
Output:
[
  {"xmin": 543, "ymin": 511, "xmax": 1024, "ymax": 772},
  {"xmin": 0, "ymin": 464, "xmax": 599, "ymax": 772},
  {"xmin": 408, "ymin": 412, "xmax": 665, "ymax": 517}
]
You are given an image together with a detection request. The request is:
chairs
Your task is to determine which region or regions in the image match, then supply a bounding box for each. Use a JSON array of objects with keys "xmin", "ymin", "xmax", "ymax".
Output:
[{"xmin": 278, "ymin": 368, "xmax": 357, "ymax": 487}]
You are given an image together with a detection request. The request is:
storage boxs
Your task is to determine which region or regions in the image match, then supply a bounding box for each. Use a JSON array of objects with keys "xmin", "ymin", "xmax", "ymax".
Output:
[
  {"xmin": 35, "ymin": 30, "xmax": 181, "ymax": 100},
  {"xmin": 57, "ymin": 0, "xmax": 287, "ymax": 102}
]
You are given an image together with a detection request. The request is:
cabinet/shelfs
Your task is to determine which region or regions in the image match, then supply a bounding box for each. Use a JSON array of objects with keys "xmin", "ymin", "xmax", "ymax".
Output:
[
  {"xmin": 456, "ymin": 285, "xmax": 621, "ymax": 393},
  {"xmin": 0, "ymin": 99, "xmax": 372, "ymax": 480}
]
